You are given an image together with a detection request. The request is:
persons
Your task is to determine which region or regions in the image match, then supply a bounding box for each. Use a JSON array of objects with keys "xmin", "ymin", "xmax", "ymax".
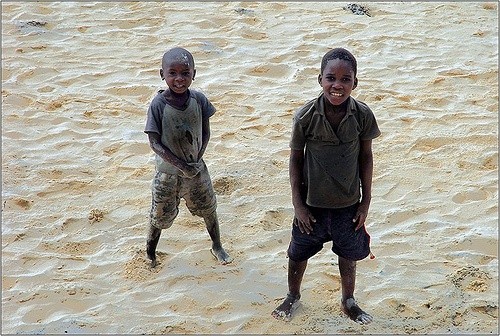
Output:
[
  {"xmin": 142, "ymin": 48, "xmax": 234, "ymax": 268},
  {"xmin": 271, "ymin": 47, "xmax": 381, "ymax": 325}
]
